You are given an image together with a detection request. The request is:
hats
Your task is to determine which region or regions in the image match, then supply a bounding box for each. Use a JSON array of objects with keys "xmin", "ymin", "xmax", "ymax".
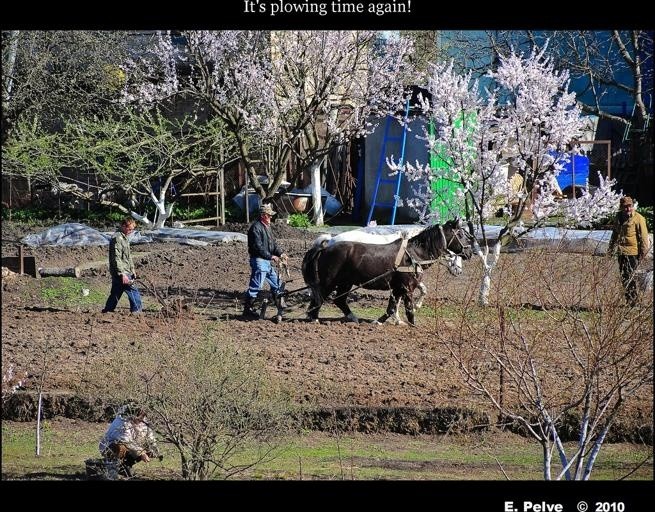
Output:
[{"xmin": 259, "ymin": 205, "xmax": 277, "ymax": 216}]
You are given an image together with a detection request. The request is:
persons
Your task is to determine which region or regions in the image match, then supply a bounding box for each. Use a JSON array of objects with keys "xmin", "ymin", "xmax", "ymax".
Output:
[
  {"xmin": 242, "ymin": 204, "xmax": 289, "ymax": 316},
  {"xmin": 99, "ymin": 402, "xmax": 159, "ymax": 475},
  {"xmin": 607, "ymin": 197, "xmax": 648, "ymax": 307},
  {"xmin": 102, "ymin": 216, "xmax": 142, "ymax": 313}
]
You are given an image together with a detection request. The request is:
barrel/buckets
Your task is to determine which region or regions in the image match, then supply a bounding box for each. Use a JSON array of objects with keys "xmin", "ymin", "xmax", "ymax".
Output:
[
  {"xmin": 84, "ymin": 458, "xmax": 121, "ymax": 481},
  {"xmin": 634, "ymin": 262, "xmax": 653, "ymax": 294},
  {"xmin": 303, "ymin": 182, "xmax": 343, "ymax": 217}
]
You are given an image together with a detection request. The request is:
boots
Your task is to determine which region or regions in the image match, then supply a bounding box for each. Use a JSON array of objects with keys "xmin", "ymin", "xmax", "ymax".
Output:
[
  {"xmin": 273, "ymin": 293, "xmax": 297, "ymax": 315},
  {"xmin": 244, "ymin": 297, "xmax": 259, "ymax": 318}
]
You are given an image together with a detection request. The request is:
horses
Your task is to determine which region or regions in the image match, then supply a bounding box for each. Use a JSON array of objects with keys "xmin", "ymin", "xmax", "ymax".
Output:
[
  {"xmin": 300, "ymin": 215, "xmax": 473, "ymax": 328},
  {"xmin": 308, "ymin": 226, "xmax": 463, "ymax": 325}
]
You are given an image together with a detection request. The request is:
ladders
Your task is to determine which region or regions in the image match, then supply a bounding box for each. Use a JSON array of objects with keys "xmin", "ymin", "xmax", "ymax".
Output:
[{"xmin": 366, "ymin": 97, "xmax": 411, "ymax": 225}]
